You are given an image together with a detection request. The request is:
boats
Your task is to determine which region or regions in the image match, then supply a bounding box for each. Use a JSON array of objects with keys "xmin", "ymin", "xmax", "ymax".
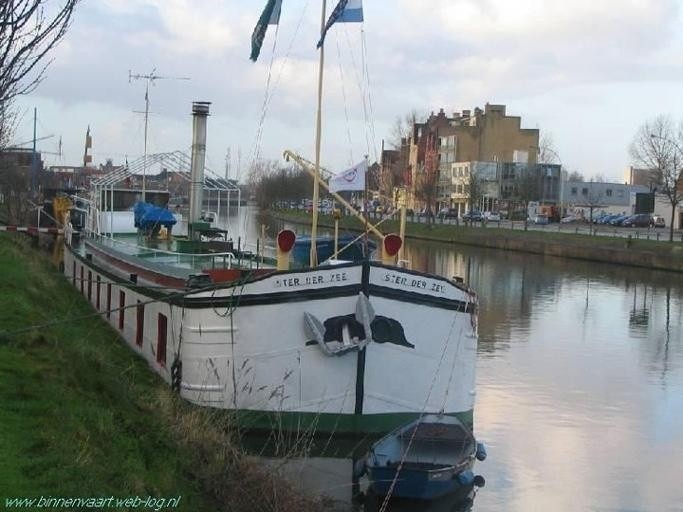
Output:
[
  {"xmin": 64, "ymin": 101, "xmax": 479, "ymax": 435},
  {"xmin": 366, "ymin": 413, "xmax": 477, "ymax": 501},
  {"xmin": 291, "ymin": 234, "xmax": 377, "ymax": 266}
]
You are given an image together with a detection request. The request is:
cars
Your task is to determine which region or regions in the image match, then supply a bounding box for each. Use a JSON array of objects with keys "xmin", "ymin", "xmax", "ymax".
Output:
[
  {"xmin": 526, "ymin": 214, "xmax": 548, "ymax": 225},
  {"xmin": 301, "ymin": 198, "xmax": 501, "ymax": 222},
  {"xmin": 596, "ymin": 214, "xmax": 665, "ymax": 228}
]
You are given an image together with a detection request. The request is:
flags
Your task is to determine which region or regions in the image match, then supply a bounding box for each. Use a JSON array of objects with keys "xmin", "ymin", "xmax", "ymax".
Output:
[
  {"xmin": 315, "ymin": 1, "xmax": 366, "ymax": 50},
  {"xmin": 247, "ymin": 0, "xmax": 283, "ymax": 63},
  {"xmin": 326, "ymin": 153, "xmax": 369, "ymax": 193}
]
